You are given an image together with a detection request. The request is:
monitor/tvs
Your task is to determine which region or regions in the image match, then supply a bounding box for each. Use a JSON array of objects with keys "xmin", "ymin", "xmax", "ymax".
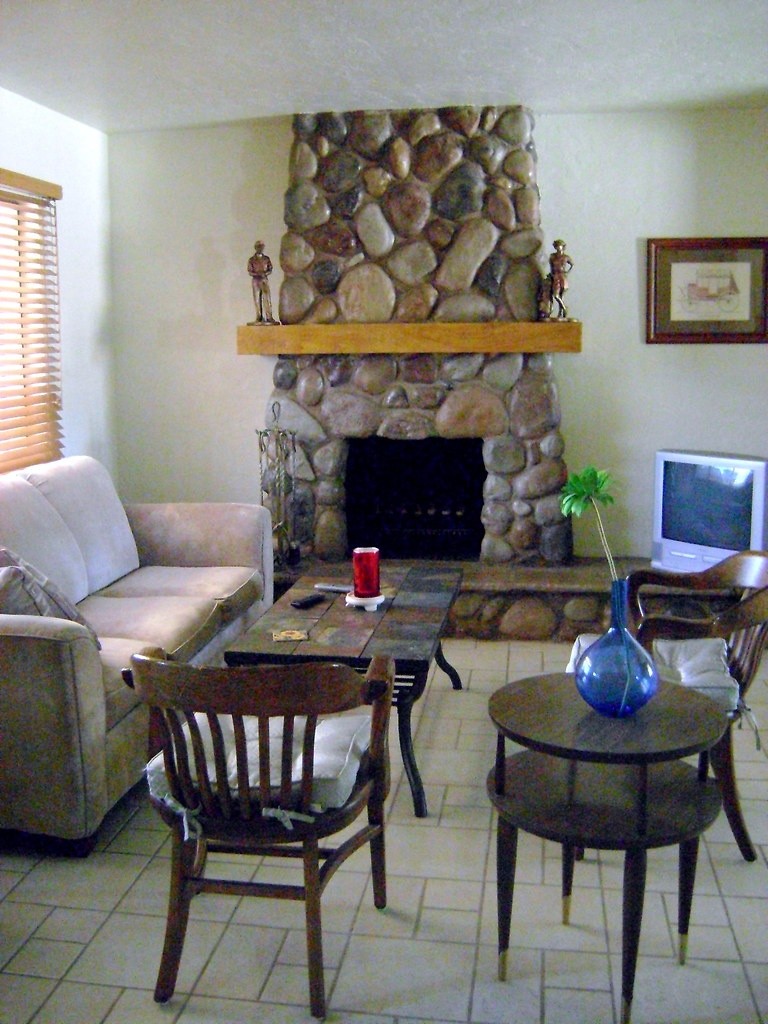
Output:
[{"xmin": 650, "ymin": 448, "xmax": 768, "ymax": 574}]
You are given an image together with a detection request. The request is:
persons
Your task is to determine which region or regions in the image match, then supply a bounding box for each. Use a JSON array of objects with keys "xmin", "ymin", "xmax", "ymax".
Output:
[
  {"xmin": 247, "ymin": 241, "xmax": 280, "ymax": 326},
  {"xmin": 549, "ymin": 240, "xmax": 574, "ymax": 317}
]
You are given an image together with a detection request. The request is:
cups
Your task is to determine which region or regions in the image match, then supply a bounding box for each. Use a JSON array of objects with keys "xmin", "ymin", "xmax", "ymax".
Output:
[{"xmin": 353, "ymin": 547, "xmax": 380, "ymax": 598}]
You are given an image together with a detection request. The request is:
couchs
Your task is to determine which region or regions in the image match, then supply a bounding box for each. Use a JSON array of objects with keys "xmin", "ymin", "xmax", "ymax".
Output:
[{"xmin": 0, "ymin": 454, "xmax": 275, "ymax": 858}]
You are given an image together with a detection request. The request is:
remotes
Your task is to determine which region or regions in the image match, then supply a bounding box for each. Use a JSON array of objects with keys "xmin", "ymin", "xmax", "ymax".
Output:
[
  {"xmin": 314, "ymin": 582, "xmax": 354, "ymax": 593},
  {"xmin": 291, "ymin": 593, "xmax": 325, "ymax": 610}
]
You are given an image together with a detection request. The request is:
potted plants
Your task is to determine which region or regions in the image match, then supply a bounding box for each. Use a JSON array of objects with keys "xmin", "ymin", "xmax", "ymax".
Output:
[{"xmin": 556, "ymin": 464, "xmax": 664, "ymax": 723}]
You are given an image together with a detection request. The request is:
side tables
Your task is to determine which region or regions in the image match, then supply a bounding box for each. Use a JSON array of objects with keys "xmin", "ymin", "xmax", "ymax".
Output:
[{"xmin": 482, "ymin": 671, "xmax": 730, "ymax": 1024}]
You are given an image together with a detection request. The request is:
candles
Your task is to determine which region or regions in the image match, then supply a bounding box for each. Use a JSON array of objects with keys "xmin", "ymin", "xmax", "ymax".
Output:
[{"xmin": 352, "ymin": 546, "xmax": 381, "ymax": 598}]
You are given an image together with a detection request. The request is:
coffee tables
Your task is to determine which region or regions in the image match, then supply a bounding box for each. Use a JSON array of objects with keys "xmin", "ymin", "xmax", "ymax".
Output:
[{"xmin": 223, "ymin": 563, "xmax": 464, "ymax": 819}]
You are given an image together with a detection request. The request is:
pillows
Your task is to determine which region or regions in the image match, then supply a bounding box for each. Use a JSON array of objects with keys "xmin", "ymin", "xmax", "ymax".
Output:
[{"xmin": 0, "ymin": 548, "xmax": 102, "ymax": 652}]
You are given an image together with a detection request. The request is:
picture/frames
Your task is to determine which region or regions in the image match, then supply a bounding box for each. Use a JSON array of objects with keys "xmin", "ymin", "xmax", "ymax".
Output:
[{"xmin": 645, "ymin": 237, "xmax": 768, "ymax": 345}]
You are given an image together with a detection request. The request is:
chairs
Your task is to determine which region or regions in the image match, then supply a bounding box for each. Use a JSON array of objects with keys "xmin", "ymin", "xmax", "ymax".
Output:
[
  {"xmin": 121, "ymin": 648, "xmax": 398, "ymax": 1024},
  {"xmin": 625, "ymin": 550, "xmax": 768, "ymax": 862}
]
